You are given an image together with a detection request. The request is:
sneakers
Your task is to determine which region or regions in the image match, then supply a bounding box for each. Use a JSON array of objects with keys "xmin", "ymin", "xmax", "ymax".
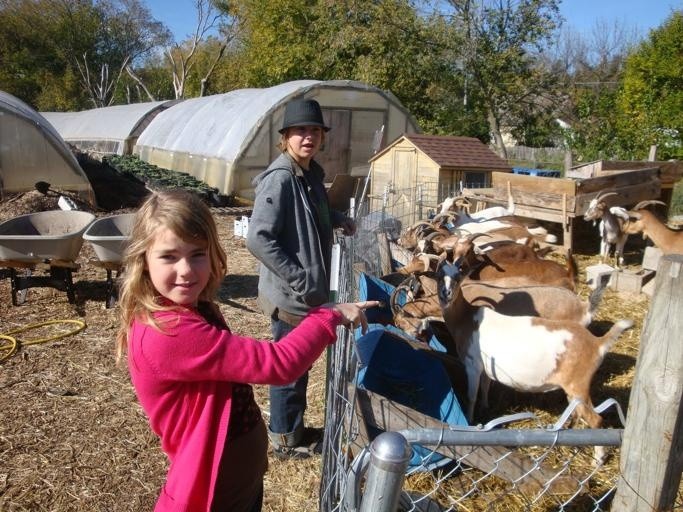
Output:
[{"xmin": 272, "ymin": 438, "xmax": 323, "ymax": 461}]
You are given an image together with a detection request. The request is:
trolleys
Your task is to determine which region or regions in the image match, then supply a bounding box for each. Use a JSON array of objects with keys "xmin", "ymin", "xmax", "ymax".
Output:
[
  {"xmin": 79, "ymin": 211, "xmax": 146, "ymax": 310},
  {"xmin": 0, "ymin": 205, "xmax": 95, "ymax": 308}
]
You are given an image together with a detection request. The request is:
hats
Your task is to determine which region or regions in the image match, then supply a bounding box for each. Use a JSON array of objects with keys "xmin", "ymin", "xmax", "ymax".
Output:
[{"xmin": 278, "ymin": 98, "xmax": 331, "ymax": 132}]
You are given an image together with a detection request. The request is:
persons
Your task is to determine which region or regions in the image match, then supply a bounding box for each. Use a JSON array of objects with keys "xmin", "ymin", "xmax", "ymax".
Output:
[
  {"xmin": 245, "ymin": 96, "xmax": 333, "ymax": 456},
  {"xmin": 114, "ymin": 188, "xmax": 380, "ymax": 512}
]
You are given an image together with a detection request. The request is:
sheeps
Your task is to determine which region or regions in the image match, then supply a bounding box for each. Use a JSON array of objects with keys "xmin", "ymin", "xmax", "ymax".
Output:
[
  {"xmin": 607, "ymin": 198, "xmax": 683, "ymax": 258},
  {"xmin": 388, "ymin": 191, "xmax": 637, "ymax": 469}
]
